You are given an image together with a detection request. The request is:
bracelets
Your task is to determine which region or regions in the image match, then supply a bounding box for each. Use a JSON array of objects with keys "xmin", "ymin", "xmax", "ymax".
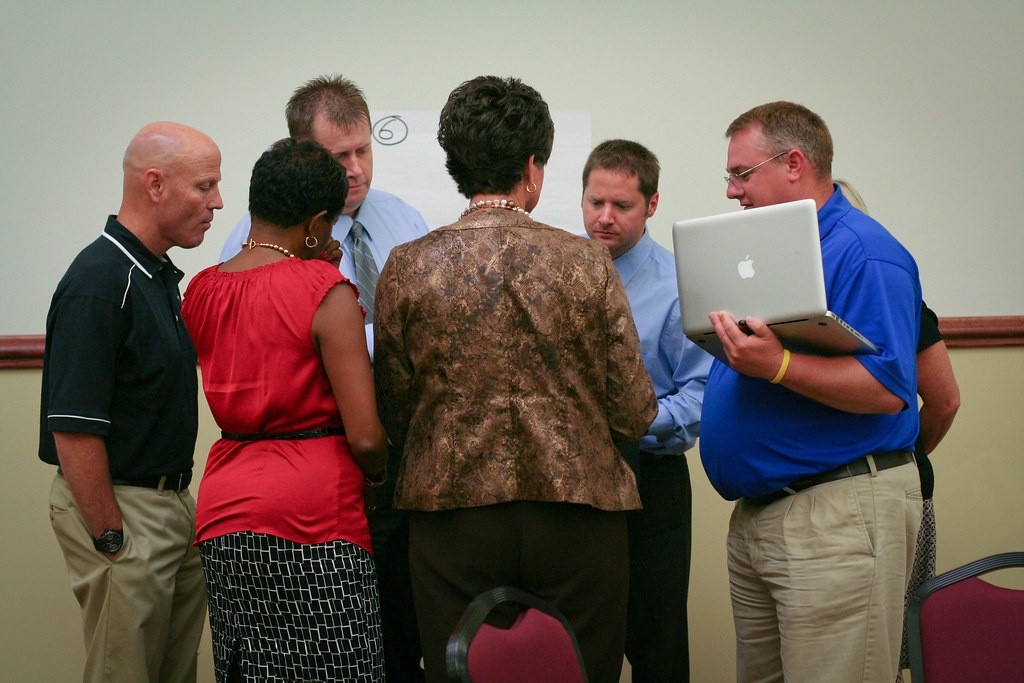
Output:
[{"xmin": 770, "ymin": 349, "xmax": 791, "ymax": 384}]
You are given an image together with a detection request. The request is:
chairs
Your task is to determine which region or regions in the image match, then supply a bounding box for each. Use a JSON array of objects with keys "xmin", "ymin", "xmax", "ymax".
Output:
[
  {"xmin": 906, "ymin": 551, "xmax": 1024, "ymax": 683},
  {"xmin": 444, "ymin": 587, "xmax": 588, "ymax": 682}
]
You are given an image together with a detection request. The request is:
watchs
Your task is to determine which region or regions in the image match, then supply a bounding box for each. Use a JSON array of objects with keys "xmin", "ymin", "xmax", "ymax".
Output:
[{"xmin": 89, "ymin": 529, "xmax": 124, "ymax": 555}]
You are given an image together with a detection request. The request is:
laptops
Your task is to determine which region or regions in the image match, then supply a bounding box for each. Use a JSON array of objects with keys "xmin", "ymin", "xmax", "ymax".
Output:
[{"xmin": 673, "ymin": 197, "xmax": 883, "ymax": 369}]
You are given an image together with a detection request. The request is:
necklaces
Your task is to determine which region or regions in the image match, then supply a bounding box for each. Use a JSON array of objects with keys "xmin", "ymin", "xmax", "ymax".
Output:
[
  {"xmin": 457, "ymin": 200, "xmax": 534, "ymax": 220},
  {"xmin": 241, "ymin": 238, "xmax": 297, "ymax": 260}
]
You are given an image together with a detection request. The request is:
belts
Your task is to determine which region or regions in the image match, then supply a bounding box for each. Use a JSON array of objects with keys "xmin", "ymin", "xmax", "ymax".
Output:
[
  {"xmin": 739, "ymin": 448, "xmax": 916, "ymax": 505},
  {"xmin": 54, "ymin": 459, "xmax": 193, "ymax": 495}
]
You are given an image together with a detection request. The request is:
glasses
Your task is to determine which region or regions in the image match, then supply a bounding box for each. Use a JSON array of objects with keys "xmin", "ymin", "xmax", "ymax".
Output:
[{"xmin": 724, "ymin": 150, "xmax": 788, "ymax": 187}]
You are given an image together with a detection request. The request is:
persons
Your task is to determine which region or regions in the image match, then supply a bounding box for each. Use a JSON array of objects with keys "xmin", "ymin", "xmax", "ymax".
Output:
[
  {"xmin": 371, "ymin": 75, "xmax": 667, "ymax": 683},
  {"xmin": 41, "ymin": 120, "xmax": 218, "ymax": 683},
  {"xmin": 696, "ymin": 99, "xmax": 921, "ymax": 683},
  {"xmin": 220, "ymin": 71, "xmax": 436, "ymax": 367},
  {"xmin": 833, "ymin": 179, "xmax": 962, "ymax": 682},
  {"xmin": 178, "ymin": 137, "xmax": 405, "ymax": 683},
  {"xmin": 575, "ymin": 140, "xmax": 716, "ymax": 683}
]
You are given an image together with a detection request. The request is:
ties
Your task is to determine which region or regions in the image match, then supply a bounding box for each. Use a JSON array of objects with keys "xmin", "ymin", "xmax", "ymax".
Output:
[{"xmin": 347, "ymin": 220, "xmax": 380, "ymax": 325}]
